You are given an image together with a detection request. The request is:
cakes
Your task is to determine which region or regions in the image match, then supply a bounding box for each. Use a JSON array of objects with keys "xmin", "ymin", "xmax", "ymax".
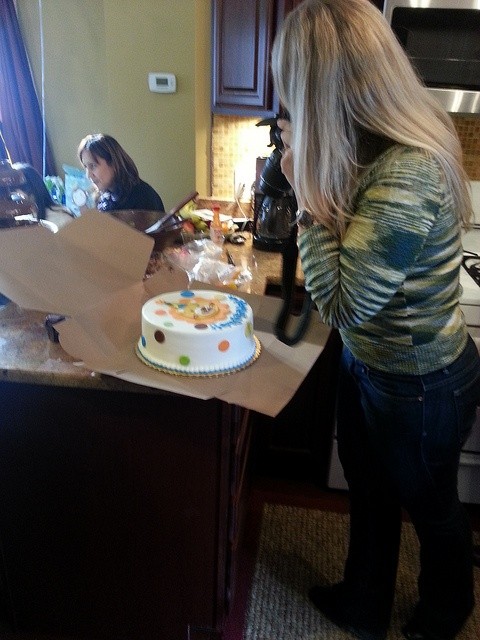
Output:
[{"xmin": 137, "ymin": 290, "xmax": 255, "ymax": 373}]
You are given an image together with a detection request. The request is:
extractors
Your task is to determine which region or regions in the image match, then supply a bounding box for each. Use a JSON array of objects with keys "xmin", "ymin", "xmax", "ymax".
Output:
[{"xmin": 381, "ymin": 2, "xmax": 479, "ymax": 115}]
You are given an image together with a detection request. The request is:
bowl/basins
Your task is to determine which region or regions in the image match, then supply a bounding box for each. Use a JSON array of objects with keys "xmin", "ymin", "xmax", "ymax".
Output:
[{"xmin": 108, "ymin": 211, "xmax": 183, "ymax": 251}]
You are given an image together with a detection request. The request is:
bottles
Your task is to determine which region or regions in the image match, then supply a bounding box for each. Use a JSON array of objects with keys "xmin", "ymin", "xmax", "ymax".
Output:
[{"xmin": 210, "ymin": 207, "xmax": 224, "ymax": 243}]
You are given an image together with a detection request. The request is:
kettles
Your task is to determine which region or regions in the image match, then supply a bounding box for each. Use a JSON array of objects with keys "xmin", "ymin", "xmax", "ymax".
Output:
[{"xmin": 253, "ymin": 195, "xmax": 303, "ymax": 251}]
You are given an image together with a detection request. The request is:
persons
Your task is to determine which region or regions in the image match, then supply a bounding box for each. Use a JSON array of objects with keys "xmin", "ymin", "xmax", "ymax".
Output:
[
  {"xmin": 10, "ymin": 160, "xmax": 77, "ymax": 230},
  {"xmin": 76, "ymin": 131, "xmax": 165, "ymax": 212},
  {"xmin": 276, "ymin": 1, "xmax": 478, "ymax": 635}
]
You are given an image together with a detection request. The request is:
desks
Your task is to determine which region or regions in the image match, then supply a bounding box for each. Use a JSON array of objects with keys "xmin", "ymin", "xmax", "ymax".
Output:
[{"xmin": 0, "ymin": 303, "xmax": 266, "ymax": 627}]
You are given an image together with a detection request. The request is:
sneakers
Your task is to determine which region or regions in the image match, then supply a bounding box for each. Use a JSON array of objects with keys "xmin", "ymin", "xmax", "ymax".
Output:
[
  {"xmin": 308, "ymin": 581, "xmax": 387, "ymax": 640},
  {"xmin": 402, "ymin": 592, "xmax": 474, "ymax": 640}
]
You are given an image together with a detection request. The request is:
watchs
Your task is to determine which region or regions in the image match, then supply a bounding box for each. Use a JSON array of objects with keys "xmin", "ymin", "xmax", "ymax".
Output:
[{"xmin": 295, "ymin": 208, "xmax": 315, "ymax": 230}]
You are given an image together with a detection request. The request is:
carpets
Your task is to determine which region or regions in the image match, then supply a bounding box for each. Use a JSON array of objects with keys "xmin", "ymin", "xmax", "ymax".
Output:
[{"xmin": 222, "ymin": 487, "xmax": 480, "ymax": 640}]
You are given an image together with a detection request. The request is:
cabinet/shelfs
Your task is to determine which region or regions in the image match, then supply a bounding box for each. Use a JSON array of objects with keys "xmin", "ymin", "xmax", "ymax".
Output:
[{"xmin": 209, "ymin": 0, "xmax": 294, "ymax": 121}]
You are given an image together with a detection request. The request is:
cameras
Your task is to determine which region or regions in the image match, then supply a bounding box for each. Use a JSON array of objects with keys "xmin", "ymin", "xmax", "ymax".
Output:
[{"xmin": 255, "ymin": 111, "xmax": 293, "ymax": 198}]
